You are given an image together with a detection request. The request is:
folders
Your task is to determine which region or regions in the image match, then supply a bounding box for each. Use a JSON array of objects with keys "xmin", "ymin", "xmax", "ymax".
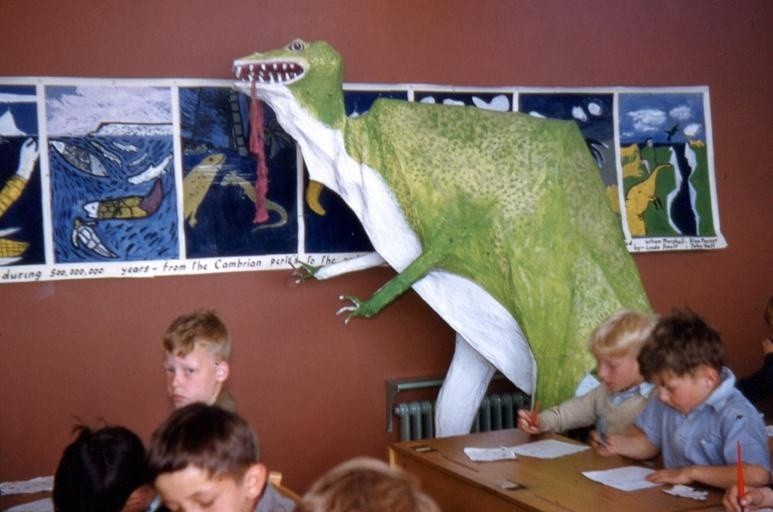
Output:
[{"xmin": 385, "ymin": 374, "xmax": 531, "ymax": 440}]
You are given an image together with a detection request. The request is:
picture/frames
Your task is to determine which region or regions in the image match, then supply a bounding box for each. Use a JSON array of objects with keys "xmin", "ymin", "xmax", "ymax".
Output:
[{"xmin": 387, "ymin": 430, "xmax": 716, "ymax": 512}]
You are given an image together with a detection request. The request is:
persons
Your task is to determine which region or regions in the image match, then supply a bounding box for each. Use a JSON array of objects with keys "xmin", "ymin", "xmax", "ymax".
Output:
[
  {"xmin": 51, "ymin": 416, "xmax": 172, "ymax": 511},
  {"xmin": 164, "ymin": 308, "xmax": 238, "ymax": 416},
  {"xmin": 589, "ymin": 302, "xmax": 772, "ymax": 492},
  {"xmin": 719, "ymin": 482, "xmax": 773, "ymax": 512},
  {"xmin": 295, "ymin": 454, "xmax": 439, "ymax": 511},
  {"xmin": 516, "ymin": 309, "xmax": 664, "ymax": 468},
  {"xmin": 733, "ymin": 293, "xmax": 772, "ymax": 420},
  {"xmin": 146, "ymin": 401, "xmax": 299, "ymax": 511}
]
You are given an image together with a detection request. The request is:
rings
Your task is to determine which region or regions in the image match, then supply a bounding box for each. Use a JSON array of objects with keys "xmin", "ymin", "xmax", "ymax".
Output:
[
  {"xmin": 530, "ymin": 400, "xmax": 542, "ymax": 429},
  {"xmin": 736, "ymin": 441, "xmax": 745, "ymax": 512}
]
